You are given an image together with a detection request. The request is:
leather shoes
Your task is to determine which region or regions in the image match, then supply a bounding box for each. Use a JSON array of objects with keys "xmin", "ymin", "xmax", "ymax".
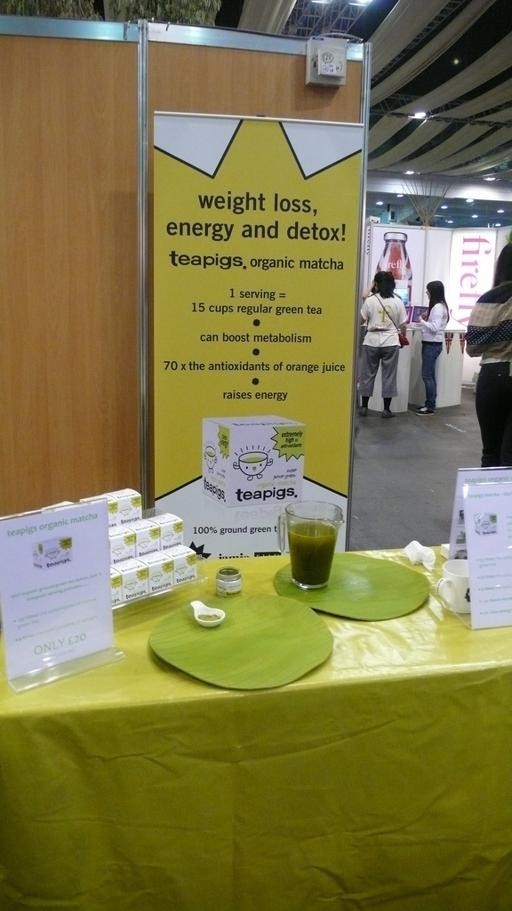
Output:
[
  {"xmin": 359, "ymin": 406, "xmax": 368, "ymax": 416},
  {"xmin": 383, "ymin": 409, "xmax": 392, "ymax": 417}
]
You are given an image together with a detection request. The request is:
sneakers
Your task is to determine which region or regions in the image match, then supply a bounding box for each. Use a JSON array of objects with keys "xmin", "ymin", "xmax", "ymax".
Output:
[{"xmin": 416, "ymin": 405, "xmax": 434, "ymax": 415}]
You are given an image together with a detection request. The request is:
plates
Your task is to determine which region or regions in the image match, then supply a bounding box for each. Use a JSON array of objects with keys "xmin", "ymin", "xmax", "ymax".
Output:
[{"xmin": 189, "ymin": 600, "xmax": 225, "ymax": 627}]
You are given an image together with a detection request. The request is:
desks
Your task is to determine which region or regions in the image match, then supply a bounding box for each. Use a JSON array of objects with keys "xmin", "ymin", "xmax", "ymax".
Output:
[{"xmin": 2, "ymin": 541, "xmax": 512, "ymax": 911}]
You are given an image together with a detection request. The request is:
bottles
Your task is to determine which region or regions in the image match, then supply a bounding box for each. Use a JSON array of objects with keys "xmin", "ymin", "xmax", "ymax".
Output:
[
  {"xmin": 376, "ymin": 232, "xmax": 411, "ymax": 312},
  {"xmin": 215, "ymin": 567, "xmax": 243, "ymax": 597}
]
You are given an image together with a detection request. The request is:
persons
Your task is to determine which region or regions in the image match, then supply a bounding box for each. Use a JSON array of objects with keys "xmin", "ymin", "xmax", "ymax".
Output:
[
  {"xmin": 466, "ymin": 243, "xmax": 512, "ymax": 467},
  {"xmin": 415, "ymin": 281, "xmax": 450, "ymax": 417},
  {"xmin": 358, "ymin": 271, "xmax": 407, "ymax": 418}
]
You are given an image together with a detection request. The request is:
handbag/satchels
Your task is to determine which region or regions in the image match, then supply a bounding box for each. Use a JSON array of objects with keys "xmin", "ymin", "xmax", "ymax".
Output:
[{"xmin": 399, "ymin": 332, "xmax": 409, "ymax": 345}]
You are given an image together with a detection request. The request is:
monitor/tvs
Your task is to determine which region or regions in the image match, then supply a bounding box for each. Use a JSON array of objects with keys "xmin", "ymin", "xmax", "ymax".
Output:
[
  {"xmin": 405, "ymin": 306, "xmax": 412, "ymax": 324},
  {"xmin": 412, "ymin": 306, "xmax": 430, "ymax": 322}
]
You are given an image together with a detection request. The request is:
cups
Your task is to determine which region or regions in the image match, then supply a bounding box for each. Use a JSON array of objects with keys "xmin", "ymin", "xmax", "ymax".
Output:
[
  {"xmin": 434, "ymin": 560, "xmax": 471, "ymax": 613},
  {"xmin": 283, "ymin": 501, "xmax": 343, "ymax": 590}
]
